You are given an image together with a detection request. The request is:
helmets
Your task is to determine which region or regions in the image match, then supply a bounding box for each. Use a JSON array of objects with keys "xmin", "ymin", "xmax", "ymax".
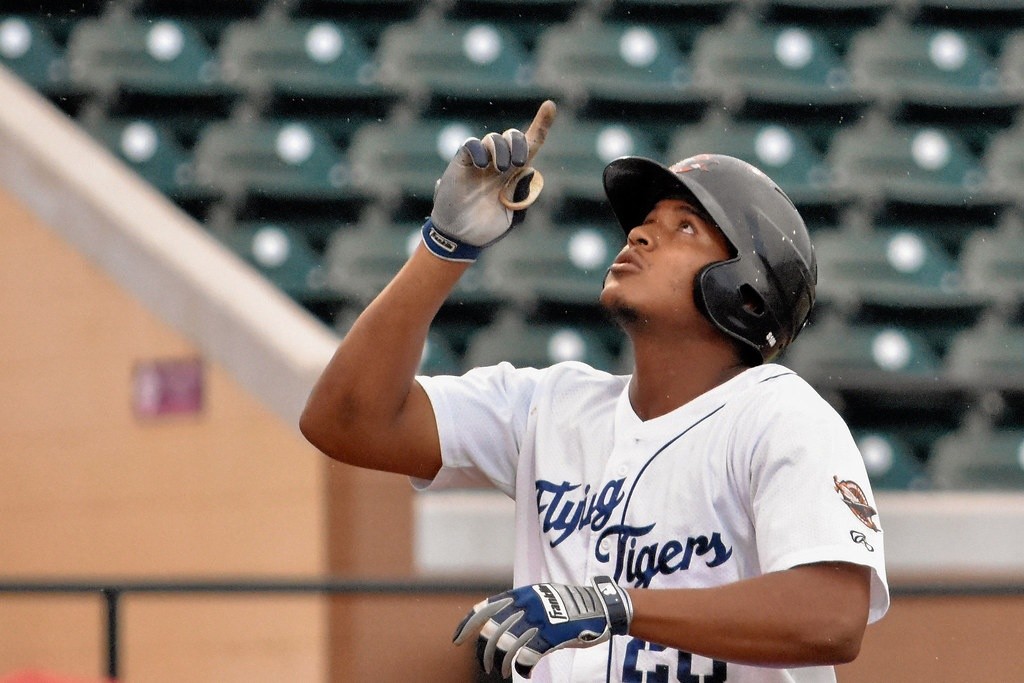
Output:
[{"xmin": 603, "ymin": 152, "xmax": 818, "ymax": 364}]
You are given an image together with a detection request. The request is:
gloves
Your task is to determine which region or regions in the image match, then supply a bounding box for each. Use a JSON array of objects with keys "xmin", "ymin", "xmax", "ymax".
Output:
[
  {"xmin": 420, "ymin": 100, "xmax": 556, "ymax": 262},
  {"xmin": 452, "ymin": 577, "xmax": 631, "ymax": 679}
]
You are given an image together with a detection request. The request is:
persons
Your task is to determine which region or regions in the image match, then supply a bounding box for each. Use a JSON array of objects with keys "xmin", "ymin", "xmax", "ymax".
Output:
[{"xmin": 297, "ymin": 100, "xmax": 893, "ymax": 682}]
